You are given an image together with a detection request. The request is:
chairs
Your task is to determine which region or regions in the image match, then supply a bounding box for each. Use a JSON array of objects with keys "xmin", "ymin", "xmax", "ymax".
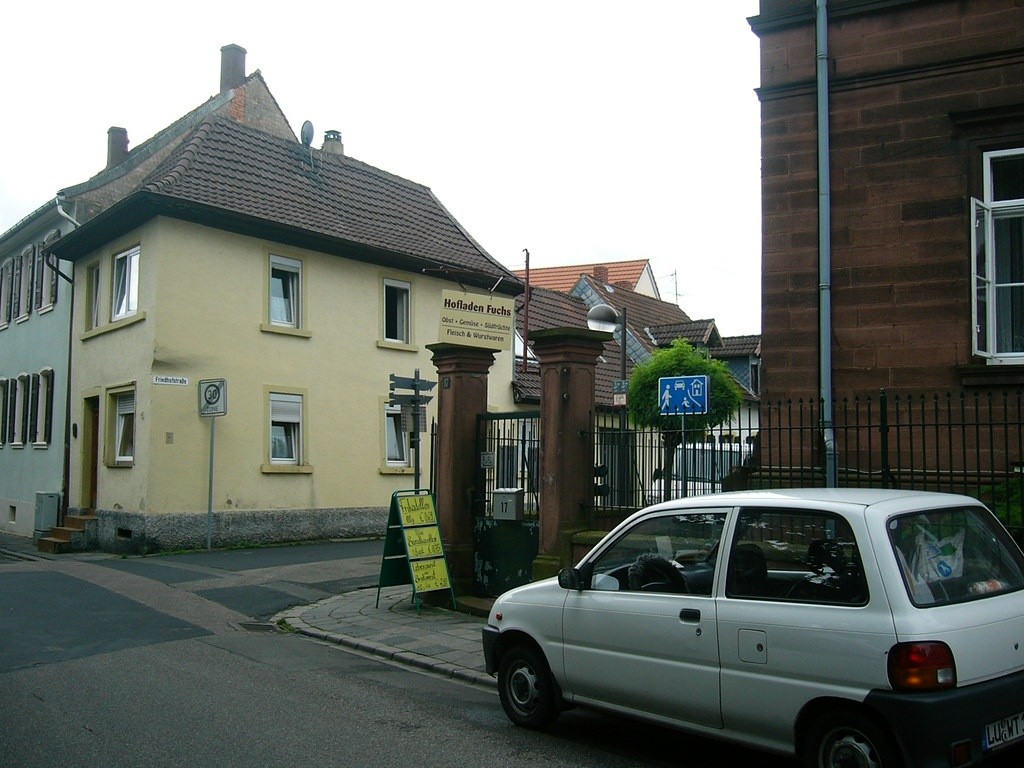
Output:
[
  {"xmin": 725, "ymin": 543, "xmax": 771, "ymax": 596},
  {"xmin": 787, "ymin": 538, "xmax": 845, "ymax": 599}
]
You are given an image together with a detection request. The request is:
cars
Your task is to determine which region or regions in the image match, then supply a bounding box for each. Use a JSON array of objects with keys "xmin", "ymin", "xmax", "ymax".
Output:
[{"xmin": 481, "ymin": 486, "xmax": 1024, "ymax": 768}]
